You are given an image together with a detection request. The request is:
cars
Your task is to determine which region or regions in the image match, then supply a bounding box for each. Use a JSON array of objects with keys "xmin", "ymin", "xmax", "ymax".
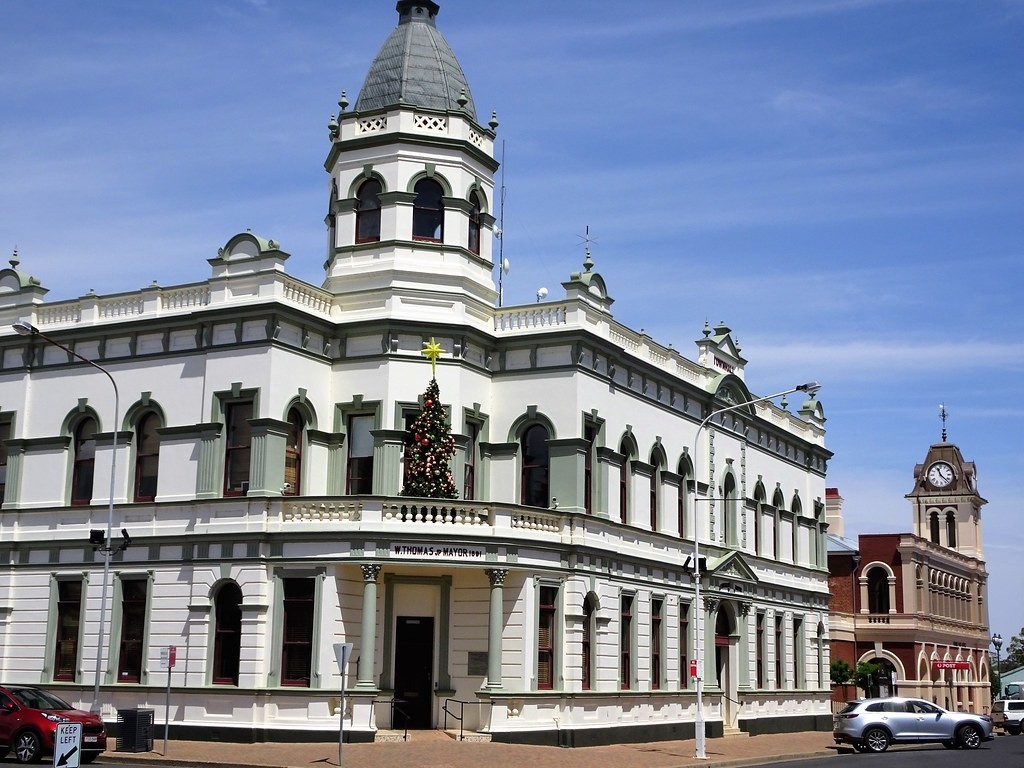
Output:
[
  {"xmin": 991, "ymin": 700, "xmax": 1024, "ymax": 736},
  {"xmin": 832, "ymin": 697, "xmax": 994, "ymax": 754},
  {"xmin": 0, "ymin": 685, "xmax": 107, "ymax": 763}
]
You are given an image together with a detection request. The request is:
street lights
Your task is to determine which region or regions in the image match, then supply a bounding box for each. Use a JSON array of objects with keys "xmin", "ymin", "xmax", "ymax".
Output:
[
  {"xmin": 691, "ymin": 380, "xmax": 822, "ymax": 760},
  {"xmin": 11, "ymin": 320, "xmax": 119, "ymax": 718},
  {"xmin": 992, "ymin": 633, "xmax": 1003, "ymax": 700}
]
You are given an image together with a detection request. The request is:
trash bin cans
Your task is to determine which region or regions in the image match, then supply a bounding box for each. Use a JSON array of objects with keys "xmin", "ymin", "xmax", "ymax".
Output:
[{"xmin": 115, "ymin": 707, "xmax": 155, "ymax": 753}]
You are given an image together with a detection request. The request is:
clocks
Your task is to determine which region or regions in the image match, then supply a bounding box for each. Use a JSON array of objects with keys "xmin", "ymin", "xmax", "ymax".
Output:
[
  {"xmin": 963, "ymin": 459, "xmax": 977, "ymax": 491},
  {"xmin": 923, "ymin": 460, "xmax": 959, "ymax": 488}
]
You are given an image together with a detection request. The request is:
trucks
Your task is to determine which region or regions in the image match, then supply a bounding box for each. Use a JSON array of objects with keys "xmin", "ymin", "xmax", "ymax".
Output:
[{"xmin": 1004, "ymin": 681, "xmax": 1024, "ymax": 700}]
